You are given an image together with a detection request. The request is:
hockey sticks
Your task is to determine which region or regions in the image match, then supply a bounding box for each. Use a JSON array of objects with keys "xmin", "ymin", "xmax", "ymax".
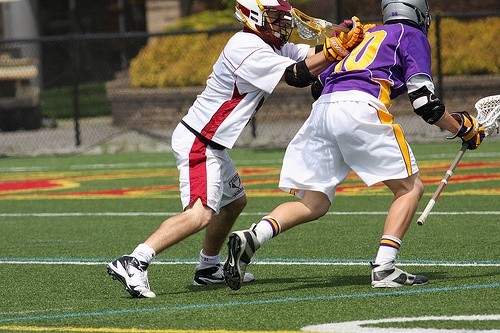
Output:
[
  {"xmin": 417, "ymin": 95, "xmax": 500, "ymax": 226},
  {"xmin": 292, "ymin": 8, "xmax": 351, "ymax": 39}
]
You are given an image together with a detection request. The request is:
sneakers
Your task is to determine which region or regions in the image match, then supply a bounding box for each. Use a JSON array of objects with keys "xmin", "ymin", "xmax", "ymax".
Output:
[
  {"xmin": 106, "ymin": 255, "xmax": 156, "ymax": 298},
  {"xmin": 369, "ymin": 260, "xmax": 430, "ymax": 289},
  {"xmin": 193, "ymin": 261, "xmax": 255, "ymax": 285},
  {"xmin": 222, "ymin": 222, "xmax": 261, "ymax": 291}
]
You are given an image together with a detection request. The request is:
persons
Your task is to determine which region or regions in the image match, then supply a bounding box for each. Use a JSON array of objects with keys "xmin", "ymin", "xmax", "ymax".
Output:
[
  {"xmin": 108, "ymin": 0, "xmax": 366, "ymax": 297},
  {"xmin": 223, "ymin": 0, "xmax": 486, "ymax": 290}
]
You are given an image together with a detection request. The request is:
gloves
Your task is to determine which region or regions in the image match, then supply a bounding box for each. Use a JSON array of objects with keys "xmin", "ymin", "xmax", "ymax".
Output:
[
  {"xmin": 323, "ymin": 16, "xmax": 364, "ymax": 64},
  {"xmin": 441, "ymin": 110, "xmax": 485, "ymax": 150}
]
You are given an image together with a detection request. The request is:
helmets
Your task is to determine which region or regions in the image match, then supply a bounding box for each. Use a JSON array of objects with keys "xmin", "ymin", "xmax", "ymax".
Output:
[
  {"xmin": 381, "ymin": 0, "xmax": 432, "ymax": 35},
  {"xmin": 233, "ymin": 0, "xmax": 296, "ymax": 51}
]
